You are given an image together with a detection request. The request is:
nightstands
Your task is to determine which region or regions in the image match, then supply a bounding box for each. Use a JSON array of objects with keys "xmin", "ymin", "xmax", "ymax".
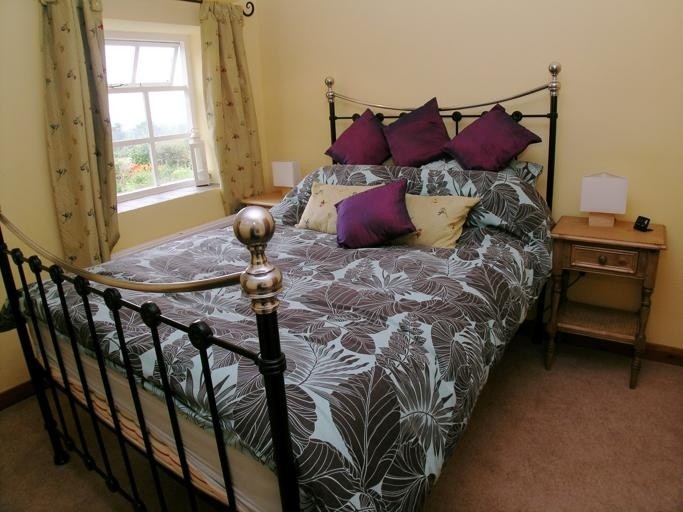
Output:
[
  {"xmin": 240, "ymin": 190, "xmax": 282, "ymax": 208},
  {"xmin": 544, "ymin": 216, "xmax": 668, "ymax": 389}
]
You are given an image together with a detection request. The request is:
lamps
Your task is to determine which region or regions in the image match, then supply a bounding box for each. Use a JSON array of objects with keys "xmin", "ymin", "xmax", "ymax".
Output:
[
  {"xmin": 189, "ymin": 127, "xmax": 209, "ymax": 187},
  {"xmin": 272, "ymin": 161, "xmax": 301, "ymax": 196},
  {"xmin": 580, "ymin": 172, "xmax": 628, "ymax": 228}
]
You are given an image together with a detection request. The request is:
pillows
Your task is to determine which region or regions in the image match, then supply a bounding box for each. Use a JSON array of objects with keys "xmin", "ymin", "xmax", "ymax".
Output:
[
  {"xmin": 269, "ymin": 160, "xmax": 559, "ymax": 242},
  {"xmin": 443, "ymin": 104, "xmax": 541, "ymax": 171},
  {"xmin": 295, "ymin": 181, "xmax": 478, "ymax": 250},
  {"xmin": 324, "ymin": 107, "xmax": 391, "ymax": 165},
  {"xmin": 378, "ymin": 96, "xmax": 450, "ymax": 167},
  {"xmin": 334, "ymin": 178, "xmax": 417, "ymax": 249}
]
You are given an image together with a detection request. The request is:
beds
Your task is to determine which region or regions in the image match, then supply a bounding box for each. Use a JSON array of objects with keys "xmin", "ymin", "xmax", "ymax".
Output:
[{"xmin": 0, "ymin": 62, "xmax": 560, "ymax": 512}]
